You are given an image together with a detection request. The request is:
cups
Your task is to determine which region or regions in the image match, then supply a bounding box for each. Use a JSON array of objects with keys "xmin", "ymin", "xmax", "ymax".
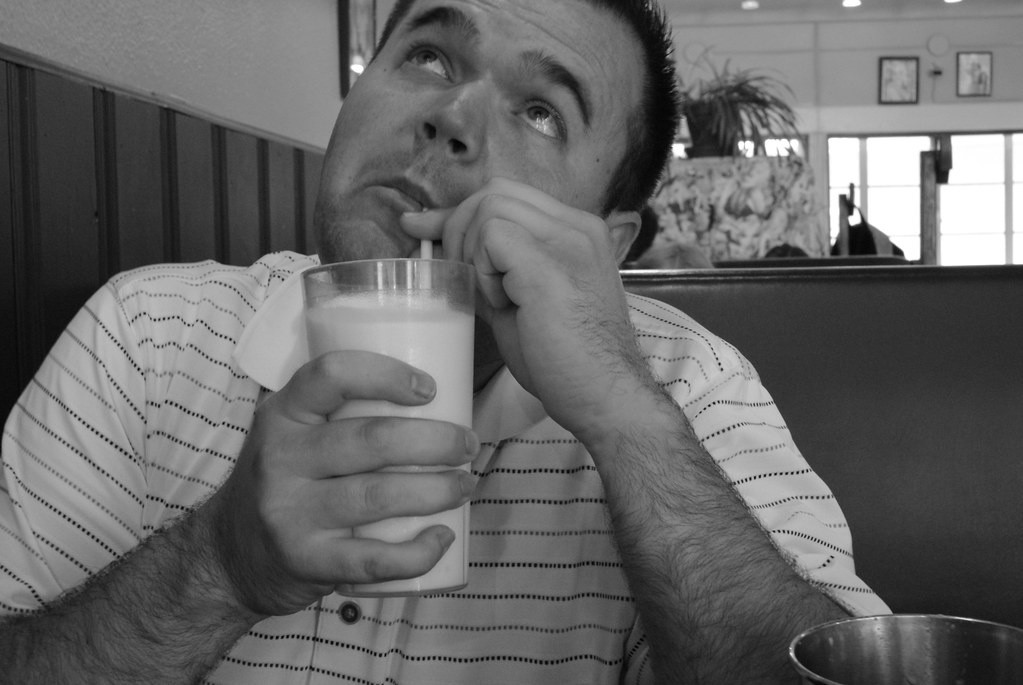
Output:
[
  {"xmin": 299, "ymin": 257, "xmax": 476, "ymax": 599},
  {"xmin": 789, "ymin": 614, "xmax": 1023, "ymax": 685}
]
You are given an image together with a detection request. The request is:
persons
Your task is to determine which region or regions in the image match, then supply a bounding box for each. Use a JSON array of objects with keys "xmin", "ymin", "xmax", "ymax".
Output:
[
  {"xmin": 1, "ymin": 0, "xmax": 894, "ymax": 685},
  {"xmin": 632, "ymin": 222, "xmax": 907, "ymax": 270}
]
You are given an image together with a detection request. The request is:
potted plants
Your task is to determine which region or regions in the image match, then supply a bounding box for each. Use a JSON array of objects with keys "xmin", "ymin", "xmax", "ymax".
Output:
[{"xmin": 673, "ymin": 41, "xmax": 807, "ymax": 168}]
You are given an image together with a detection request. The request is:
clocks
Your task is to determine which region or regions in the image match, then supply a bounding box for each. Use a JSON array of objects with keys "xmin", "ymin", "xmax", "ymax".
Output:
[{"xmin": 927, "ymin": 33, "xmax": 951, "ymax": 56}]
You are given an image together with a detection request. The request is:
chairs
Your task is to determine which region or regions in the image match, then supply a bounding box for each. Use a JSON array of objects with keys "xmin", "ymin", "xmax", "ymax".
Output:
[{"xmin": 45, "ymin": 266, "xmax": 1023, "ymax": 630}]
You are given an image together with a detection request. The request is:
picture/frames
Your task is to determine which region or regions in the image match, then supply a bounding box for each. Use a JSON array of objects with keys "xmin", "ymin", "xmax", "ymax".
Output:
[
  {"xmin": 879, "ymin": 57, "xmax": 919, "ymax": 104},
  {"xmin": 337, "ymin": 0, "xmax": 376, "ymax": 100},
  {"xmin": 956, "ymin": 51, "xmax": 993, "ymax": 97}
]
